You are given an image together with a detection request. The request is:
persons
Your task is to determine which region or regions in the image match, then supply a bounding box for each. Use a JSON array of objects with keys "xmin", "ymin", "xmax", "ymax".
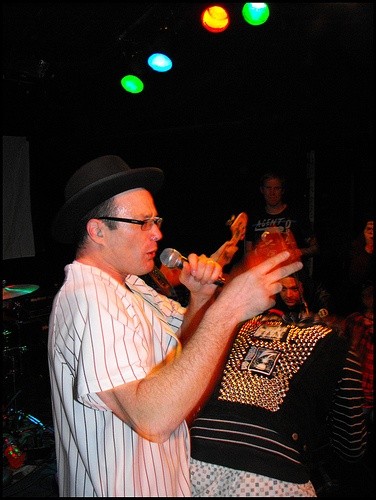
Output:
[
  {"xmin": 49, "ymin": 154, "xmax": 305, "ymax": 498},
  {"xmin": 229, "ymin": 172, "xmax": 376, "ymax": 455}
]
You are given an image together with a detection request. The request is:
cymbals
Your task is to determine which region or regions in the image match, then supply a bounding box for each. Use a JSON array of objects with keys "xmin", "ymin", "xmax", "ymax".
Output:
[{"xmin": 3, "ymin": 285, "xmax": 39, "ymax": 299}]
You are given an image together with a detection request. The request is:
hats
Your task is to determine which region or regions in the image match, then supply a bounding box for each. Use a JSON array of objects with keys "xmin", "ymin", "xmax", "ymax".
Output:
[{"xmin": 55, "ymin": 155, "xmax": 166, "ymax": 233}]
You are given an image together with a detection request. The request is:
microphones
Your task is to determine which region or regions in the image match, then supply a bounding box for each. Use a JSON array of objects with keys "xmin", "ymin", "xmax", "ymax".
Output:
[{"xmin": 160, "ymin": 248, "xmax": 227, "ymax": 288}]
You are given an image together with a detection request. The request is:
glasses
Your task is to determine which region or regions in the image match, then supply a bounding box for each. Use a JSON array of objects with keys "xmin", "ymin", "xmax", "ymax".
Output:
[{"xmin": 94, "ymin": 217, "xmax": 164, "ymax": 231}]
